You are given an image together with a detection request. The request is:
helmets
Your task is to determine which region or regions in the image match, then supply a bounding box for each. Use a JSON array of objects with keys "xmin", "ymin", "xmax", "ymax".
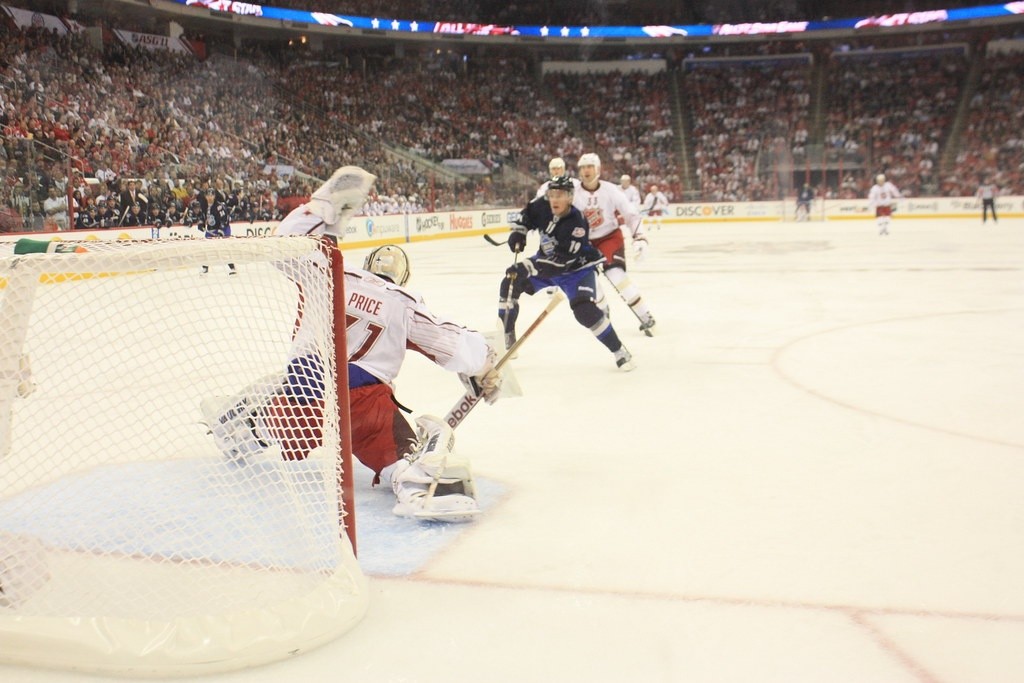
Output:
[
  {"xmin": 546, "ymin": 175, "xmax": 574, "ymax": 205},
  {"xmin": 578, "ymin": 153, "xmax": 601, "ymax": 177},
  {"xmin": 621, "ymin": 175, "xmax": 631, "ymax": 182},
  {"xmin": 549, "ymin": 157, "xmax": 565, "ymax": 178},
  {"xmin": 362, "ymin": 245, "xmax": 410, "ymax": 287}
]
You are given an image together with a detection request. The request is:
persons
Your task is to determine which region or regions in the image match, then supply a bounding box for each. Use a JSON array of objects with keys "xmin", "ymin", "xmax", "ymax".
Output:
[
  {"xmin": 643, "ymin": 185, "xmax": 669, "ymax": 231},
  {"xmin": 201, "ymin": 166, "xmax": 503, "ymax": 523},
  {"xmin": 868, "ymin": 174, "xmax": 900, "ymax": 236},
  {"xmin": 572, "ymin": 152, "xmax": 656, "ymax": 338},
  {"xmin": 0, "ymin": 0, "xmax": 1024, "ymax": 230},
  {"xmin": 616, "ymin": 174, "xmax": 650, "ymax": 248},
  {"xmin": 498, "ymin": 176, "xmax": 634, "ymax": 369},
  {"xmin": 197, "ymin": 188, "xmax": 239, "ymax": 279},
  {"xmin": 975, "ymin": 176, "xmax": 997, "ymax": 224},
  {"xmin": 531, "ymin": 157, "xmax": 580, "ymax": 294},
  {"xmin": 794, "ymin": 182, "xmax": 815, "ymax": 221}
]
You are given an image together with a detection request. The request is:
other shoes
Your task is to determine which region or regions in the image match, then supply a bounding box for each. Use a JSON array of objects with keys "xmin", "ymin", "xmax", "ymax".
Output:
[{"xmin": 393, "ymin": 493, "xmax": 478, "ymax": 523}]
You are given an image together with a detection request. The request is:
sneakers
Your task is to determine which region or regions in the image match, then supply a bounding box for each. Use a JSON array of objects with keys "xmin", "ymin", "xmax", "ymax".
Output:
[
  {"xmin": 638, "ymin": 311, "xmax": 656, "ymax": 333},
  {"xmin": 505, "ymin": 329, "xmax": 518, "ymax": 359},
  {"xmin": 611, "ymin": 344, "xmax": 635, "ymax": 372}
]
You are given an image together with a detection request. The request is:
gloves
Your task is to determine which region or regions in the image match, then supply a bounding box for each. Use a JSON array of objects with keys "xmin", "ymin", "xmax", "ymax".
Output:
[
  {"xmin": 508, "ymin": 224, "xmax": 528, "ymax": 252},
  {"xmin": 505, "ymin": 258, "xmax": 538, "ymax": 280},
  {"xmin": 474, "ymin": 359, "xmax": 501, "ymax": 395}
]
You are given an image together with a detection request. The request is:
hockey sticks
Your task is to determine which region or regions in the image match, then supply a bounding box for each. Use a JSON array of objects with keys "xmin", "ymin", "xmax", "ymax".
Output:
[
  {"xmin": 503, "ymin": 240, "xmax": 520, "ymax": 333},
  {"xmin": 483, "ymin": 234, "xmax": 508, "ymax": 246},
  {"xmin": 118, "ymin": 205, "xmax": 130, "ymax": 228},
  {"xmin": 595, "ymin": 264, "xmax": 653, "ymax": 337},
  {"xmin": 202, "ymin": 226, "xmax": 221, "ymax": 237},
  {"xmin": 227, "ymin": 206, "xmax": 235, "ymax": 219},
  {"xmin": 182, "ymin": 206, "xmax": 188, "ymax": 222},
  {"xmin": 444, "ymin": 293, "xmax": 564, "ymax": 432},
  {"xmin": 616, "ymin": 197, "xmax": 658, "ymax": 219},
  {"xmin": 138, "ymin": 193, "xmax": 149, "ymax": 224},
  {"xmin": 259, "ymin": 195, "xmax": 262, "ymax": 216}
]
